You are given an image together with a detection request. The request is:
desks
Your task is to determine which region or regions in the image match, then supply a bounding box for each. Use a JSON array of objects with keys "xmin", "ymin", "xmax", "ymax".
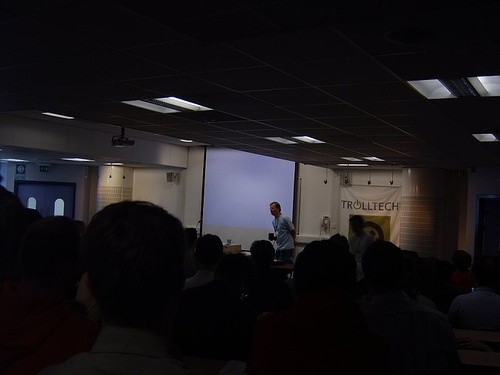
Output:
[
  {"xmin": 458, "ymin": 349, "xmax": 500, "ymax": 367},
  {"xmin": 453, "ymin": 328, "xmax": 500, "ymax": 345}
]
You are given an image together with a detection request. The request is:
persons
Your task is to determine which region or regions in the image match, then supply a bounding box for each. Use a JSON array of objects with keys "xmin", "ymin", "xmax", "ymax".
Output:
[
  {"xmin": 206, "ymin": 233, "xmax": 500, "ymax": 375},
  {"xmin": 270, "ymin": 202, "xmax": 296, "ymax": 279},
  {"xmin": 0, "ymin": 159, "xmax": 223, "ymax": 375},
  {"xmin": 348, "ymin": 215, "xmax": 374, "ymax": 280}
]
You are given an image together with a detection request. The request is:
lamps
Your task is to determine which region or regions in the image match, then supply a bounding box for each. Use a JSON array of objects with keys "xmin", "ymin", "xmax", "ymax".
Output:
[
  {"xmin": 324, "ymin": 167, "xmax": 327, "ymax": 184},
  {"xmin": 389, "ymin": 166, "xmax": 393, "ymax": 185},
  {"xmin": 368, "ymin": 161, "xmax": 372, "ymax": 185}
]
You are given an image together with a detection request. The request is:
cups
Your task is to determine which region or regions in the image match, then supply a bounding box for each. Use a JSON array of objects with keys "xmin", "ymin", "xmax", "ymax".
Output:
[
  {"xmin": 269, "ymin": 233, "xmax": 274, "ymax": 240},
  {"xmin": 227, "ymin": 239, "xmax": 231, "ymax": 243}
]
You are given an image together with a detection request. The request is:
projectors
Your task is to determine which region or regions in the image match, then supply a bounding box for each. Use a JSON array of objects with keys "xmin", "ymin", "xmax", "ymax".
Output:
[{"xmin": 112, "ymin": 138, "xmax": 135, "ymax": 148}]
